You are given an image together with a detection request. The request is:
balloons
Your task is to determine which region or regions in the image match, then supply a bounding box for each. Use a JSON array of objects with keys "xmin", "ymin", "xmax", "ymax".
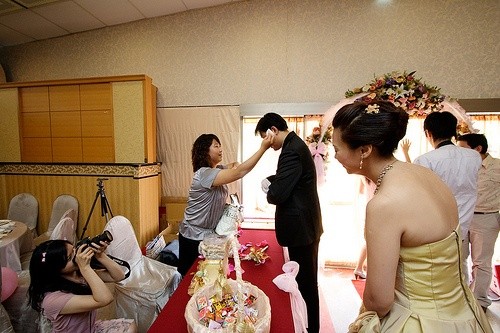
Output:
[{"xmin": 1, "ymin": 266, "xmax": 19, "ymax": 303}]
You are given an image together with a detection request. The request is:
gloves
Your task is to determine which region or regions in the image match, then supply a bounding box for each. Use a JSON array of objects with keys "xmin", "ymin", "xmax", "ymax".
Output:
[{"xmin": 262, "ymin": 178, "xmax": 271, "ymax": 191}]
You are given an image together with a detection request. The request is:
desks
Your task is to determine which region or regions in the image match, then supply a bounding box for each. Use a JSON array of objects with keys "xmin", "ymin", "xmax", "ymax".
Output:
[
  {"xmin": 0, "ymin": 219, "xmax": 34, "ymax": 272},
  {"xmin": 147, "ymin": 226, "xmax": 296, "ymax": 333}
]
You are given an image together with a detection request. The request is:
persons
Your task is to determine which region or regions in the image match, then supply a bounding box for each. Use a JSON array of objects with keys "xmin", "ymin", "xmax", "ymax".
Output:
[
  {"xmin": 332, "ymin": 100, "xmax": 494, "ymax": 333},
  {"xmin": 412, "ymin": 111, "xmax": 481, "ymax": 288},
  {"xmin": 177, "ymin": 132, "xmax": 275, "ymax": 279},
  {"xmin": 355, "ymin": 139, "xmax": 413, "ymax": 280},
  {"xmin": 255, "ymin": 112, "xmax": 324, "ymax": 333},
  {"xmin": 457, "ymin": 132, "xmax": 500, "ymax": 314},
  {"xmin": 26, "ymin": 240, "xmax": 125, "ymax": 333}
]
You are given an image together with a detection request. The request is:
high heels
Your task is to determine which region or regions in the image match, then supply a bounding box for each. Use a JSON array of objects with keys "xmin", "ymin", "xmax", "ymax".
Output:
[{"xmin": 354, "ymin": 270, "xmax": 366, "ymax": 280}]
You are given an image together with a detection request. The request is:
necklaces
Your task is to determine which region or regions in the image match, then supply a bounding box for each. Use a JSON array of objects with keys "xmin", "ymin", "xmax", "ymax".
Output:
[{"xmin": 374, "ymin": 160, "xmax": 397, "ymax": 194}]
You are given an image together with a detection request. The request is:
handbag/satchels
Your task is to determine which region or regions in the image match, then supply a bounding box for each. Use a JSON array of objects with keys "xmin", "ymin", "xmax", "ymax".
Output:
[
  {"xmin": 146, "ymin": 234, "xmax": 166, "ymax": 261},
  {"xmin": 216, "ymin": 193, "xmax": 244, "ymax": 236}
]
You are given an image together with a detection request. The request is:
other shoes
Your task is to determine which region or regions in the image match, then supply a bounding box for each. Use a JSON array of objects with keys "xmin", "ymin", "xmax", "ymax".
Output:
[{"xmin": 482, "ymin": 306, "xmax": 487, "ymax": 313}]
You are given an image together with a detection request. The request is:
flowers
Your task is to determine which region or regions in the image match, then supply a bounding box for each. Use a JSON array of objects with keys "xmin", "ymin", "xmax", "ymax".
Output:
[{"xmin": 308, "ymin": 71, "xmax": 474, "ymax": 167}]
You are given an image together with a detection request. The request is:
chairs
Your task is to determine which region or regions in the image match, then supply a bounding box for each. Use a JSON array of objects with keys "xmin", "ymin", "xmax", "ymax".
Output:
[
  {"xmin": 0, "ymin": 192, "xmax": 79, "ymax": 333},
  {"xmin": 98, "ymin": 216, "xmax": 183, "ymax": 333}
]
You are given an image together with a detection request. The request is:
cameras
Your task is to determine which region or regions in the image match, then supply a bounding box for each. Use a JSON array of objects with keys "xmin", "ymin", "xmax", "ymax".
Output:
[{"xmin": 73, "ymin": 230, "xmax": 114, "ymax": 266}]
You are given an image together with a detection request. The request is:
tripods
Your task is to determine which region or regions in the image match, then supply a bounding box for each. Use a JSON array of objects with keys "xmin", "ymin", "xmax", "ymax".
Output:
[{"xmin": 79, "ymin": 188, "xmax": 113, "ymax": 240}]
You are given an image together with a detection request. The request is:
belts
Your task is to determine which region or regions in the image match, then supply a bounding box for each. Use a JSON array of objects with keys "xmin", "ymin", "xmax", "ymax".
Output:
[{"xmin": 474, "ymin": 211, "xmax": 499, "ymax": 214}]
